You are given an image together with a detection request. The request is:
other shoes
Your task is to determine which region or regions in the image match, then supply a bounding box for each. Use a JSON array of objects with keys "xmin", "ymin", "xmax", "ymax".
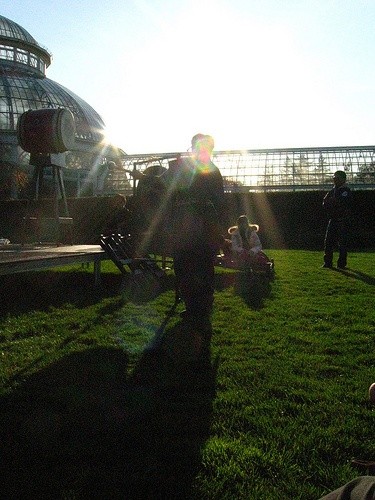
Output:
[
  {"xmin": 179, "ymin": 310, "xmax": 210, "ymax": 331},
  {"xmin": 324, "ymin": 263, "xmax": 333, "ymax": 268},
  {"xmin": 338, "ymin": 265, "xmax": 346, "ymax": 269}
]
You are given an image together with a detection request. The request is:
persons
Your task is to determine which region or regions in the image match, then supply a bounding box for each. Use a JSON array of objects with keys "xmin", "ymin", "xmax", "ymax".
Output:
[
  {"xmin": 321, "ymin": 171, "xmax": 353, "ymax": 269},
  {"xmin": 105, "ymin": 195, "xmax": 140, "ymax": 237},
  {"xmin": 228, "ymin": 216, "xmax": 271, "ymax": 266},
  {"xmin": 109, "ymin": 134, "xmax": 225, "ymax": 322}
]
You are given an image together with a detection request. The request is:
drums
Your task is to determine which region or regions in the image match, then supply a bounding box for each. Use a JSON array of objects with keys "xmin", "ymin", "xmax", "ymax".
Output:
[
  {"xmin": 136, "ymin": 166, "xmax": 173, "ymax": 224},
  {"xmin": 17, "ymin": 107, "xmax": 75, "ymax": 154}
]
